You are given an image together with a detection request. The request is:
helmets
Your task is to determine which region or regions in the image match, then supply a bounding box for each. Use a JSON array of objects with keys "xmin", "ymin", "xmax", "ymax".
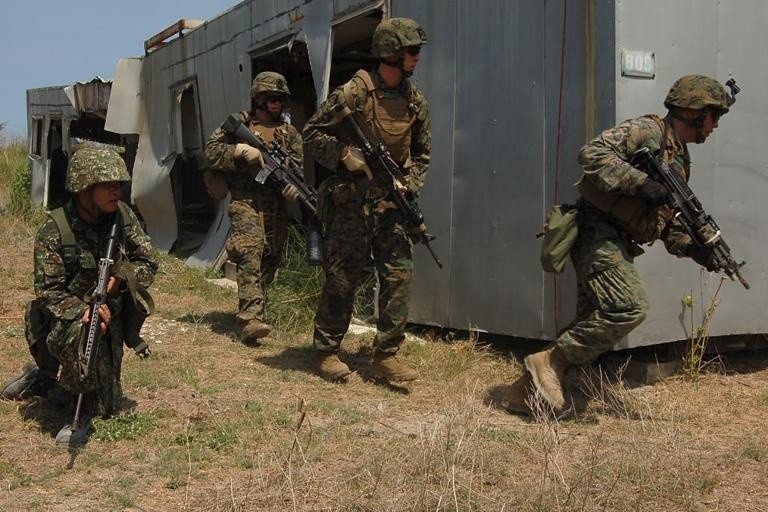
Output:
[
  {"xmin": 250, "ymin": 71, "xmax": 291, "ymax": 98},
  {"xmin": 65, "ymin": 148, "xmax": 132, "ymax": 194},
  {"xmin": 371, "ymin": 18, "xmax": 427, "ymax": 63},
  {"xmin": 664, "ymin": 74, "xmax": 729, "ymax": 114}
]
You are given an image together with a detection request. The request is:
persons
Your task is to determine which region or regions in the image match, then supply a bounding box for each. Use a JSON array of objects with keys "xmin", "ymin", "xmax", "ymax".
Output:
[
  {"xmin": 495, "ymin": 71, "xmax": 740, "ymax": 422},
  {"xmin": 1, "ymin": 145, "xmax": 163, "ymax": 453},
  {"xmin": 301, "ymin": 16, "xmax": 433, "ymax": 388},
  {"xmin": 203, "ymin": 69, "xmax": 305, "ymax": 346}
]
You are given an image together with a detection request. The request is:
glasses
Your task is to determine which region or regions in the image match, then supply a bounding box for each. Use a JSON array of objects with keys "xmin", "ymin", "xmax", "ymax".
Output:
[
  {"xmin": 408, "ymin": 46, "xmax": 420, "ymax": 55},
  {"xmin": 265, "ymin": 94, "xmax": 287, "ymax": 103}
]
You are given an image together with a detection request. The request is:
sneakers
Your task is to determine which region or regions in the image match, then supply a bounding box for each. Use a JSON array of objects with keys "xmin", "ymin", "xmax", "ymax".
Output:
[
  {"xmin": 54, "ymin": 413, "xmax": 92, "ymax": 447},
  {"xmin": 307, "ymin": 352, "xmax": 349, "ymax": 382},
  {"xmin": 502, "ymin": 345, "xmax": 587, "ymax": 419},
  {"xmin": 1, "ymin": 362, "xmax": 41, "ymax": 399},
  {"xmin": 240, "ymin": 319, "xmax": 271, "ymax": 343},
  {"xmin": 370, "ymin": 350, "xmax": 417, "ymax": 381}
]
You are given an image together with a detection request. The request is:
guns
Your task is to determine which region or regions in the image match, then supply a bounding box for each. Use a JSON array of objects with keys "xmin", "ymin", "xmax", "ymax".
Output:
[
  {"xmin": 634, "ymin": 146, "xmax": 751, "ymax": 291},
  {"xmin": 70, "ymin": 224, "xmax": 124, "ymax": 432},
  {"xmin": 310, "ymin": 101, "xmax": 443, "ymax": 271},
  {"xmin": 220, "ymin": 113, "xmax": 319, "ymax": 212}
]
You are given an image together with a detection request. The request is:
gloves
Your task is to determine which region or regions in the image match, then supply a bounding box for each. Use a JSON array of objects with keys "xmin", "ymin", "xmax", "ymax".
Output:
[
  {"xmin": 282, "ymin": 184, "xmax": 300, "ymax": 202},
  {"xmin": 376, "ymin": 200, "xmax": 399, "ymax": 210},
  {"xmin": 235, "ymin": 144, "xmax": 266, "ymax": 171},
  {"xmin": 639, "ymin": 179, "xmax": 669, "ymax": 206},
  {"xmin": 342, "ymin": 147, "xmax": 373, "ymax": 181},
  {"xmin": 685, "ymin": 243, "xmax": 720, "ymax": 273}
]
